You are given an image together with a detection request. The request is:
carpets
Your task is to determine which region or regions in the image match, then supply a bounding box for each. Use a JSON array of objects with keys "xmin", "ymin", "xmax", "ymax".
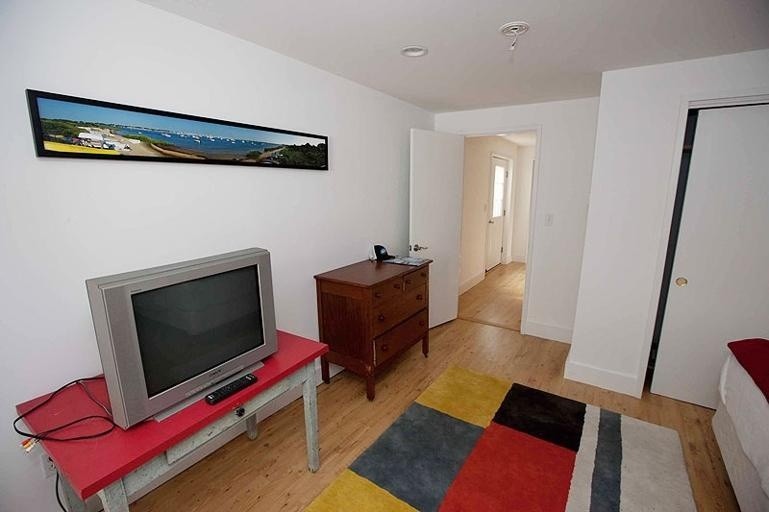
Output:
[{"xmin": 301, "ymin": 366, "xmax": 699, "ymax": 511}]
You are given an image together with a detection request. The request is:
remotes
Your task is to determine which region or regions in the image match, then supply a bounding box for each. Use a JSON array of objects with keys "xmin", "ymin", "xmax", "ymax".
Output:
[{"xmin": 206, "ymin": 373, "xmax": 257, "ymax": 405}]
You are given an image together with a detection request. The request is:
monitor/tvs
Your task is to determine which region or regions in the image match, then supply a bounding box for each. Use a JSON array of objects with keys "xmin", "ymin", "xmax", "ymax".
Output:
[{"xmin": 85, "ymin": 246, "xmax": 279, "ymax": 431}]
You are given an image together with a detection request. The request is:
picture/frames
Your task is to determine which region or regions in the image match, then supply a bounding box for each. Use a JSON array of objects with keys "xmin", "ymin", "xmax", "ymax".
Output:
[{"xmin": 25, "ymin": 89, "xmax": 329, "ymax": 171}]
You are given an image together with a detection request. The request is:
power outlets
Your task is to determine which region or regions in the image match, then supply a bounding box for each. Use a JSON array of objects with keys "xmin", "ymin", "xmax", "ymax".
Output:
[{"xmin": 41, "ymin": 454, "xmax": 58, "ymax": 479}]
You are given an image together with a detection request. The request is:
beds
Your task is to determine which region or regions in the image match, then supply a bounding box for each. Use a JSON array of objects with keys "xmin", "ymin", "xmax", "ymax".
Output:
[{"xmin": 711, "ymin": 337, "xmax": 769, "ymax": 512}]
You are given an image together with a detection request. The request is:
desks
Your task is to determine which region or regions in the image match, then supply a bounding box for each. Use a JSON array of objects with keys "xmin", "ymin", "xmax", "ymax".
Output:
[{"xmin": 16, "ymin": 329, "xmax": 330, "ymax": 512}]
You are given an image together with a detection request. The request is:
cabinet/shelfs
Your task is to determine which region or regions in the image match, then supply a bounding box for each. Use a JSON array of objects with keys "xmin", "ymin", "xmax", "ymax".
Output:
[{"xmin": 313, "ymin": 255, "xmax": 433, "ymax": 401}]
